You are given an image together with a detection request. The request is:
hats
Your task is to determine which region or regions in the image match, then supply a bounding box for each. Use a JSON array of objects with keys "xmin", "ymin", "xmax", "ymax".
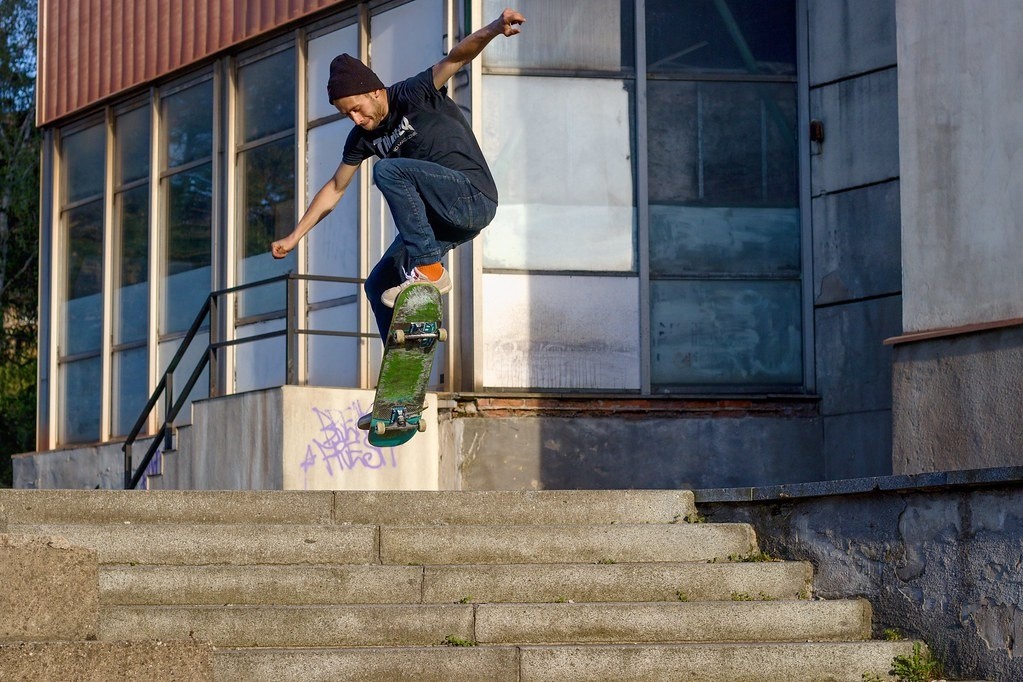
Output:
[{"xmin": 327, "ymin": 53, "xmax": 385, "ymax": 105}]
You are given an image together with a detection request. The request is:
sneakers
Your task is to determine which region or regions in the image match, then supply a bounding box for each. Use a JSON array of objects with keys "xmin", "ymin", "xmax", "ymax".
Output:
[
  {"xmin": 381, "ymin": 265, "xmax": 453, "ymax": 308},
  {"xmin": 358, "ymin": 398, "xmax": 428, "ymax": 430}
]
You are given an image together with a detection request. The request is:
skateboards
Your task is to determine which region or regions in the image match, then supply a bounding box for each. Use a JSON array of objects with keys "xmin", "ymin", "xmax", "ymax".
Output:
[{"xmin": 367, "ymin": 279, "xmax": 448, "ymax": 449}]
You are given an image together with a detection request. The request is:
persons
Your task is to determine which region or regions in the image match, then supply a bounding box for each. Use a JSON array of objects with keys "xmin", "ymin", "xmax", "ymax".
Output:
[{"xmin": 271, "ymin": 8, "xmax": 526, "ymax": 430}]
